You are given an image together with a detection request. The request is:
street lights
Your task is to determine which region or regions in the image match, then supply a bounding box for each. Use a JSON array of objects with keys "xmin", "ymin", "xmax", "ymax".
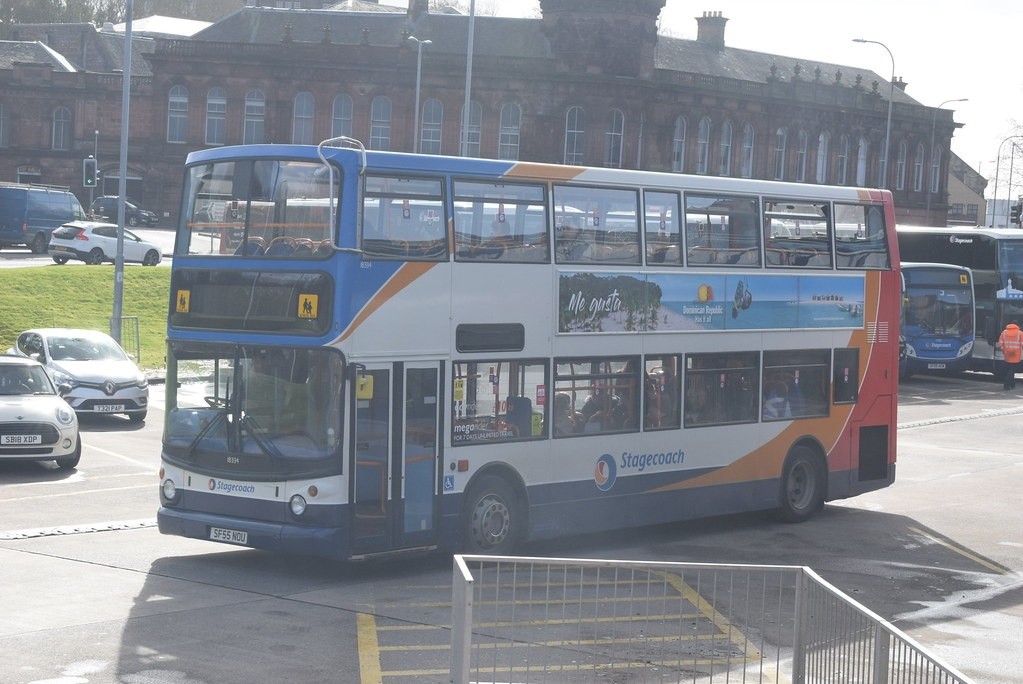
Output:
[
  {"xmin": 852, "ymin": 37, "xmax": 896, "ymax": 188},
  {"xmin": 408, "ymin": 35, "xmax": 432, "ymax": 154},
  {"xmin": 926, "ymin": 98, "xmax": 969, "ymax": 224}
]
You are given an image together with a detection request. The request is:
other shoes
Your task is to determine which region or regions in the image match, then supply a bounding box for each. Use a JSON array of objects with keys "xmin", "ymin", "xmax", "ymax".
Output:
[
  {"xmin": 1004, "ymin": 388, "xmax": 1010, "ymax": 390},
  {"xmin": 1011, "ymin": 383, "xmax": 1015, "ymax": 388}
]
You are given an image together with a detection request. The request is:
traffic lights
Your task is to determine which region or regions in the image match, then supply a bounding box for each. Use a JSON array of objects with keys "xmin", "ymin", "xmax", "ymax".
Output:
[
  {"xmin": 1011, "ymin": 204, "xmax": 1022, "ymax": 224},
  {"xmin": 83, "ymin": 159, "xmax": 97, "ymax": 187}
]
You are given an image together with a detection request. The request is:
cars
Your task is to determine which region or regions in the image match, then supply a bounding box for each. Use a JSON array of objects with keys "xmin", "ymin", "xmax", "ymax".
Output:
[
  {"xmin": 48, "ymin": 220, "xmax": 163, "ymax": 266},
  {"xmin": 5, "ymin": 327, "xmax": 149, "ymax": 420},
  {"xmin": 0, "ymin": 356, "xmax": 81, "ymax": 468},
  {"xmin": 90, "ymin": 194, "xmax": 160, "ymax": 227}
]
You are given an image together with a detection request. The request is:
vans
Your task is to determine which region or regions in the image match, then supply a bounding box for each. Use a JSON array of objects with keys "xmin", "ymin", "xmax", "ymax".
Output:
[{"xmin": 0, "ymin": 181, "xmax": 91, "ymax": 254}]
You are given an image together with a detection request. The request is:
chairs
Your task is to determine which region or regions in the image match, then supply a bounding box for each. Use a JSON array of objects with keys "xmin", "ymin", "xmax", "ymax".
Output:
[
  {"xmin": 496, "ymin": 365, "xmax": 840, "ymax": 438},
  {"xmin": 229, "ymin": 223, "xmax": 876, "ymax": 270}
]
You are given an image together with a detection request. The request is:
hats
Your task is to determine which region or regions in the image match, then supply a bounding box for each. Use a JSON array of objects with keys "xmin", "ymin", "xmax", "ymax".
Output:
[{"xmin": 1011, "ymin": 319, "xmax": 1018, "ymax": 325}]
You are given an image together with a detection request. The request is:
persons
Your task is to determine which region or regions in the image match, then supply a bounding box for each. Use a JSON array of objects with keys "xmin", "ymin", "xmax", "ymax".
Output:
[
  {"xmin": 540, "ymin": 356, "xmax": 792, "ymax": 437},
  {"xmin": 555, "ymin": 219, "xmax": 591, "ymax": 261},
  {"xmin": 490, "ymin": 221, "xmax": 512, "ymax": 240},
  {"xmin": 999, "ymin": 320, "xmax": 1023, "ymax": 390}
]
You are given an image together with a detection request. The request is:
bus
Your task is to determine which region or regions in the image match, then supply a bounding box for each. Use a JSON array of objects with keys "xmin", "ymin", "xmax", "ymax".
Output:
[{"xmin": 154, "ymin": 136, "xmax": 1023, "ymax": 568}]
